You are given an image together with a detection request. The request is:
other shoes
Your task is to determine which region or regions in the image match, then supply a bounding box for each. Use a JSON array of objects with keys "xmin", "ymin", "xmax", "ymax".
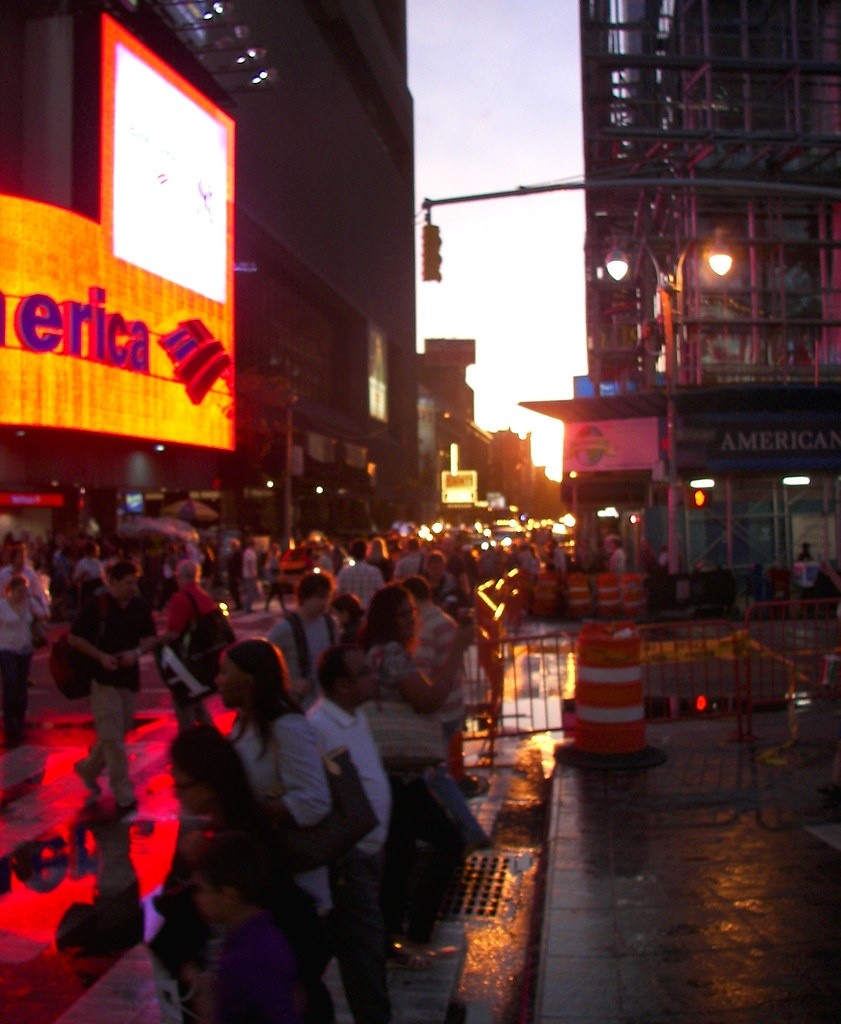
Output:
[
  {"xmin": 118, "ymin": 799, "xmax": 136, "ymax": 825},
  {"xmin": 76, "ymin": 760, "xmax": 100, "ymax": 796}
]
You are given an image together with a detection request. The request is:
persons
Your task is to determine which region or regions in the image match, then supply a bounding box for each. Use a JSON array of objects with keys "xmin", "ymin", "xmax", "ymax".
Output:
[
  {"xmin": 1, "ymin": 520, "xmax": 565, "ymax": 1024},
  {"xmin": 605, "ymin": 534, "xmax": 627, "ymax": 573},
  {"xmin": 658, "ymin": 544, "xmax": 668, "ymax": 570},
  {"xmin": 820, "ymin": 559, "xmax": 841, "ymax": 642},
  {"xmin": 797, "ymin": 543, "xmax": 813, "ymax": 561}
]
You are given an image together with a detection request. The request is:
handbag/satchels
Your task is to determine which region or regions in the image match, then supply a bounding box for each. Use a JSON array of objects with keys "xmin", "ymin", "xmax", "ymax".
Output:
[
  {"xmin": 254, "ymin": 713, "xmax": 383, "ymax": 873},
  {"xmin": 151, "ymin": 590, "xmax": 236, "ymax": 707},
  {"xmin": 49, "ymin": 632, "xmax": 92, "ymax": 699},
  {"xmin": 353, "ymin": 649, "xmax": 446, "ymax": 770}
]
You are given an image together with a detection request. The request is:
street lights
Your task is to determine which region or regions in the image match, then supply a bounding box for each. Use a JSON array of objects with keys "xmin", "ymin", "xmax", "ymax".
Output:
[
  {"xmin": 285, "ymin": 395, "xmax": 300, "ymax": 538},
  {"xmin": 606, "ymin": 232, "xmax": 733, "ymax": 572}
]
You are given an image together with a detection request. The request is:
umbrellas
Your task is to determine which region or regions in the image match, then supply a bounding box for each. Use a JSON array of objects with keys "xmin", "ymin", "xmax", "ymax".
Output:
[{"xmin": 163, "ymin": 499, "xmax": 219, "ymax": 521}]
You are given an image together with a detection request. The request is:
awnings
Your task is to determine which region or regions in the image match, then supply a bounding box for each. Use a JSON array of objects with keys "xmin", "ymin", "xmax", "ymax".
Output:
[
  {"xmin": 525, "ymin": 392, "xmax": 658, "ymax": 422},
  {"xmin": 670, "ymin": 385, "xmax": 841, "ymax": 417}
]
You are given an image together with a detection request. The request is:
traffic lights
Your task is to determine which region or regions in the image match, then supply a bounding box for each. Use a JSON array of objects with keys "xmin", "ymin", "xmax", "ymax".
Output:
[
  {"xmin": 688, "ymin": 490, "xmax": 708, "ymax": 510},
  {"xmin": 422, "ymin": 225, "xmax": 442, "ymax": 281}
]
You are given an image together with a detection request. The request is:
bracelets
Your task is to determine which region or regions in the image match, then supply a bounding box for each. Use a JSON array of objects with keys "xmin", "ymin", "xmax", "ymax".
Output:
[{"xmin": 135, "ymin": 646, "xmax": 143, "ymax": 657}]
